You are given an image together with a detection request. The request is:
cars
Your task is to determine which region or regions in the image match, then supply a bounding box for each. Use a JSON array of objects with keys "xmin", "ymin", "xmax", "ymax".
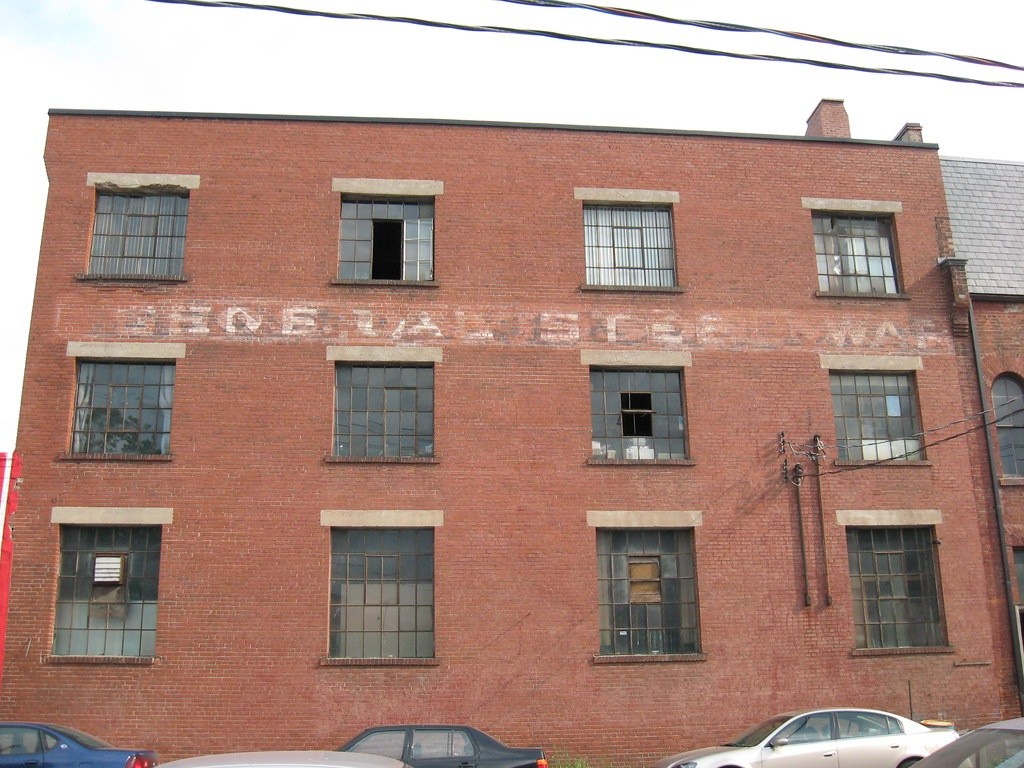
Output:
[
  {"xmin": 151, "ymin": 750, "xmax": 413, "ymax": 768},
  {"xmin": 907, "ymin": 713, "xmax": 1024, "ymax": 768},
  {"xmin": 654, "ymin": 705, "xmax": 975, "ymax": 768},
  {"xmin": 0, "ymin": 721, "xmax": 159, "ymax": 768},
  {"xmin": 329, "ymin": 724, "xmax": 550, "ymax": 768}
]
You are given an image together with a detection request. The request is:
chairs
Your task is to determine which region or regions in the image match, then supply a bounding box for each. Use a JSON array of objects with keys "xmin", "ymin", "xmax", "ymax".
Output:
[
  {"xmin": 414, "ymin": 743, "xmax": 422, "ymax": 759},
  {"xmin": 805, "ymin": 724, "xmax": 830, "ymax": 739}
]
